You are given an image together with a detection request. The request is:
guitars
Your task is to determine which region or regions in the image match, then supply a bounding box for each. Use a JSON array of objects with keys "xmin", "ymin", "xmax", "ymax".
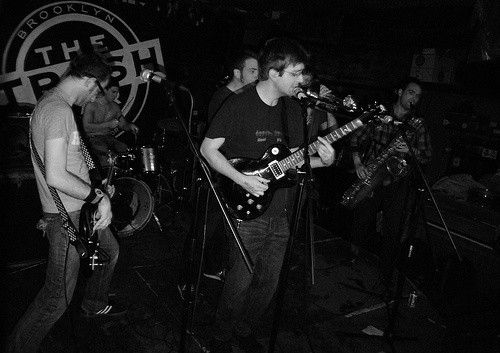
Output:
[
  {"xmin": 213, "ymin": 98, "xmax": 386, "ymax": 221},
  {"xmin": 80, "ymin": 156, "xmax": 117, "ymax": 277}
]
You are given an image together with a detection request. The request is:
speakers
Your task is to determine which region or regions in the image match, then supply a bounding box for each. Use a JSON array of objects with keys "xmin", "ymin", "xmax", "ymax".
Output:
[
  {"xmin": 0, "ymin": 102, "xmax": 36, "ymax": 181},
  {"xmin": 0, "ymin": 179, "xmax": 51, "ymax": 275}
]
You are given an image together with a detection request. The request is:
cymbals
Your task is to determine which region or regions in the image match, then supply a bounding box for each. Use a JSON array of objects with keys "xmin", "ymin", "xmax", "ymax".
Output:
[{"xmin": 155, "ymin": 116, "xmax": 188, "ymax": 131}]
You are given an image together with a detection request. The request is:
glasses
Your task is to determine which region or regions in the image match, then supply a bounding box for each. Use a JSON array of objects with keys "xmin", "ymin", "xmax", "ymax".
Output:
[
  {"xmin": 280, "ymin": 69, "xmax": 303, "ymax": 77},
  {"xmin": 95, "ymin": 79, "xmax": 106, "ymax": 99}
]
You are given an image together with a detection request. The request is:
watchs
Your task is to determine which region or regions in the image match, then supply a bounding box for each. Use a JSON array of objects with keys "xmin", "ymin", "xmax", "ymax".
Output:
[{"xmin": 91, "ymin": 188, "xmax": 105, "ymax": 205}]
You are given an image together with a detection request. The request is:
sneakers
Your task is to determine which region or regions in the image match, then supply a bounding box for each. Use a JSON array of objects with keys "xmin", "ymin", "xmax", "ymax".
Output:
[{"xmin": 80, "ymin": 302, "xmax": 129, "ymax": 320}]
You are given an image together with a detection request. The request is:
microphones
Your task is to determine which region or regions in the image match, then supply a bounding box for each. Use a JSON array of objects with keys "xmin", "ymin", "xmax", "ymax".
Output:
[
  {"xmin": 292, "ymin": 87, "xmax": 311, "ymax": 106},
  {"xmin": 384, "ymin": 115, "xmax": 403, "ymax": 125},
  {"xmin": 140, "ymin": 70, "xmax": 187, "ymax": 93}
]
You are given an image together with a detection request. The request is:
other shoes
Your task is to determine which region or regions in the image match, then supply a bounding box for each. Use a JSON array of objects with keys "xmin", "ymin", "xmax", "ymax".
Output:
[
  {"xmin": 209, "ymin": 338, "xmax": 231, "ymax": 353},
  {"xmin": 238, "ymin": 336, "xmax": 266, "ymax": 353}
]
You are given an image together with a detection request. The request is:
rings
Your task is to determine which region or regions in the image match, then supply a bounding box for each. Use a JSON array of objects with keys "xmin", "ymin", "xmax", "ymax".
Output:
[{"xmin": 136, "ymin": 128, "xmax": 138, "ymax": 131}]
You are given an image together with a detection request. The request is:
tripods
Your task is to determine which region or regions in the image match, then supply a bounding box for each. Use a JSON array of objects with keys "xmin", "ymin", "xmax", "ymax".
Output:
[{"xmin": 336, "ymin": 124, "xmax": 463, "ymax": 353}]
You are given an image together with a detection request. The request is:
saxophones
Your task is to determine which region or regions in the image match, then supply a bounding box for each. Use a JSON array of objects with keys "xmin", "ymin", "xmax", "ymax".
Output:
[{"xmin": 339, "ymin": 101, "xmax": 424, "ymax": 208}]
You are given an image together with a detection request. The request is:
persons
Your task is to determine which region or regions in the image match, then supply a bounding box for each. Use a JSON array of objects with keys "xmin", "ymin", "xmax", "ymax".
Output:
[
  {"xmin": 83, "ymin": 52, "xmax": 431, "ymax": 288},
  {"xmin": 4, "ymin": 52, "xmax": 128, "ymax": 353},
  {"xmin": 201, "ymin": 39, "xmax": 336, "ymax": 353}
]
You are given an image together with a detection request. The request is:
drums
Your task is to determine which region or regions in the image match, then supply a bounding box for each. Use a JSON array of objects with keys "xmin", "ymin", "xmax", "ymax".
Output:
[
  {"xmin": 127, "ymin": 144, "xmax": 161, "ymax": 175},
  {"xmin": 104, "ymin": 175, "xmax": 155, "ymax": 238}
]
